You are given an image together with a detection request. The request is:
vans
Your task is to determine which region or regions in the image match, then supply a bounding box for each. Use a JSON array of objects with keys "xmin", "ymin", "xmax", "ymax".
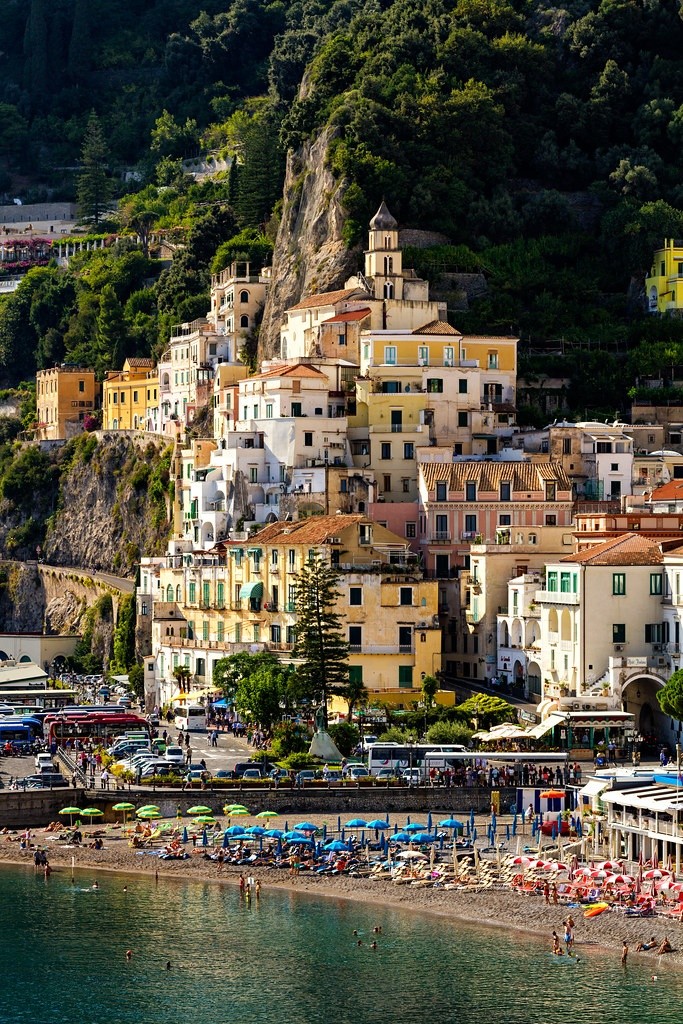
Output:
[
  {"xmin": 106, "ymin": 731, "xmax": 151, "ymax": 754},
  {"xmin": 163, "ymin": 746, "xmax": 185, "ymax": 765},
  {"xmin": 234, "ymin": 762, "xmax": 280, "ymax": 778},
  {"xmin": 17, "ymin": 773, "xmax": 69, "ymax": 787}
]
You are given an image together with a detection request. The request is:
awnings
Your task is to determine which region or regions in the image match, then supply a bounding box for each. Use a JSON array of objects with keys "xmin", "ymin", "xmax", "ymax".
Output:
[
  {"xmin": 503, "ymin": 729, "xmax": 536, "ymax": 740},
  {"xmin": 490, "ymin": 721, "xmax": 523, "ymax": 731},
  {"xmin": 487, "ymin": 728, "xmax": 511, "ymax": 737},
  {"xmin": 210, "ymin": 698, "xmax": 229, "ymax": 708},
  {"xmin": 471, "ymin": 734, "xmax": 502, "ymax": 742},
  {"xmin": 189, "ymin": 688, "xmax": 223, "ymax": 699}
]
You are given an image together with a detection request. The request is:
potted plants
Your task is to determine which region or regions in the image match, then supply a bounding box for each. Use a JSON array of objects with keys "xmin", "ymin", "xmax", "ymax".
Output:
[{"xmin": 141, "ymin": 774, "xmax": 394, "ymax": 787}]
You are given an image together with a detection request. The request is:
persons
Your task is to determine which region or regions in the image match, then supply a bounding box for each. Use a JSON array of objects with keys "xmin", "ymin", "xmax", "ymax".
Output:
[
  {"xmin": 490, "ymin": 803, "xmax": 498, "ymax": 819},
  {"xmin": 70, "ymin": 868, "xmax": 159, "ymax": 892},
  {"xmin": 106, "ymin": 820, "xmax": 196, "ymax": 858},
  {"xmin": 214, "ymin": 843, "xmax": 347, "ymax": 876},
  {"xmin": 0, "ymin": 820, "xmax": 104, "ymax": 877},
  {"xmin": 161, "ymin": 703, "xmax": 616, "ymax": 786},
  {"xmin": 240, "ymin": 872, "xmax": 261, "ymax": 900},
  {"xmin": 491, "ymin": 673, "xmax": 497, "ymax": 690},
  {"xmin": 91, "ymin": 564, "xmax": 97, "ymax": 575},
  {"xmin": 543, "ymin": 879, "xmax": 668, "ymax": 916},
  {"xmin": 352, "ymin": 879, "xmax": 673, "ymax": 963},
  {"xmin": 0, "ymin": 677, "xmax": 143, "ymax": 790},
  {"xmin": 526, "ymin": 803, "xmax": 535, "ymax": 823},
  {"xmin": 595, "ymin": 732, "xmax": 674, "ymax": 769}
]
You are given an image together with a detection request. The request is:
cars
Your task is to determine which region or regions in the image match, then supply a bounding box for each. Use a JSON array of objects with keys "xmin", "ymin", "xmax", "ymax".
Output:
[
  {"xmin": 150, "ymin": 714, "xmax": 159, "ymax": 726},
  {"xmin": 187, "ymin": 764, "xmax": 209, "ymax": 777},
  {"xmin": 243, "ymin": 769, "xmax": 342, "ymax": 781},
  {"xmin": 125, "ymin": 748, "xmax": 181, "ymax": 778},
  {"xmin": 116, "ymin": 697, "xmax": 131, "ymax": 709},
  {"xmin": 98, "ymin": 685, "xmax": 110, "ymax": 701},
  {"xmin": 59, "ymin": 672, "xmax": 102, "ymax": 685},
  {"xmin": 109, "ymin": 682, "xmax": 133, "ymax": 698},
  {"xmin": 217, "ymin": 770, "xmax": 235, "ymax": 779},
  {"xmin": 152, "ymin": 738, "xmax": 166, "ymax": 752}
]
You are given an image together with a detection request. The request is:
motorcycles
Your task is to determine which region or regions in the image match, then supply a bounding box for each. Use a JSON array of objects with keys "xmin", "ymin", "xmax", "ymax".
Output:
[{"xmin": 1, "ymin": 746, "xmax": 21, "ymax": 758}]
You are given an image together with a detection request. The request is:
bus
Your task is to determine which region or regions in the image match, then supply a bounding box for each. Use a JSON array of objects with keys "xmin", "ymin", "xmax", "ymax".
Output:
[
  {"xmin": 368, "ymin": 741, "xmax": 466, "ymax": 776},
  {"xmin": 0, "ymin": 690, "xmax": 149, "ymax": 751},
  {"xmin": 175, "ymin": 705, "xmax": 207, "ymax": 731}
]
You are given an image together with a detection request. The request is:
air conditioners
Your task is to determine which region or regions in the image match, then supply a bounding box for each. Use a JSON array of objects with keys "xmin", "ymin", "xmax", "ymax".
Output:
[
  {"xmin": 615, "ymin": 645, "xmax": 624, "ymax": 651},
  {"xmin": 419, "ymin": 620, "xmax": 429, "ymax": 627},
  {"xmin": 652, "ymin": 644, "xmax": 662, "ymax": 651},
  {"xmin": 573, "ymin": 701, "xmax": 583, "ymax": 711},
  {"xmin": 585, "ymin": 704, "xmax": 592, "ymax": 711},
  {"xmin": 657, "ymin": 657, "xmax": 667, "ymax": 665},
  {"xmin": 326, "ymin": 537, "xmax": 341, "ymax": 543}
]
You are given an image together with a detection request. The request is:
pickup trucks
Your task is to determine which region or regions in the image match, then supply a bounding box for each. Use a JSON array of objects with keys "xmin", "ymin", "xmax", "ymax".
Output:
[{"xmin": 35, "ymin": 752, "xmax": 56, "ymax": 774}]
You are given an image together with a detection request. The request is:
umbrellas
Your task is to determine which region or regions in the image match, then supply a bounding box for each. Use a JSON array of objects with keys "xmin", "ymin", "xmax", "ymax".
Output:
[
  {"xmin": 346, "ymin": 818, "xmax": 391, "ymax": 829},
  {"xmin": 285, "ymin": 813, "xmax": 345, "ymax": 841},
  {"xmin": 293, "ymin": 821, "xmax": 318, "ymax": 830},
  {"xmin": 324, "ymin": 842, "xmax": 348, "ymax": 851},
  {"xmin": 396, "ymin": 850, "xmax": 428, "ymax": 870},
  {"xmin": 182, "ymin": 826, "xmax": 208, "ymax": 845},
  {"xmin": 58, "ymin": 804, "xmax": 279, "ymax": 823},
  {"xmin": 439, "ymin": 818, "xmax": 465, "ymax": 828},
  {"xmin": 467, "ymin": 787, "xmax": 582, "ymax": 845},
  {"xmin": 390, "ymin": 823, "xmax": 435, "ymax": 842},
  {"xmin": 512, "ymin": 855, "xmax": 683, "ymax": 893},
  {"xmin": 394, "ymin": 811, "xmax": 454, "ymax": 834},
  {"xmin": 223, "ymin": 825, "xmax": 315, "ymax": 845}
]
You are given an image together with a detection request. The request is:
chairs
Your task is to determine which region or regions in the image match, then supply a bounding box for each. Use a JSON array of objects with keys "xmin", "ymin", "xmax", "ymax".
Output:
[{"xmin": 8, "ymin": 823, "xmax": 683, "ymax": 922}]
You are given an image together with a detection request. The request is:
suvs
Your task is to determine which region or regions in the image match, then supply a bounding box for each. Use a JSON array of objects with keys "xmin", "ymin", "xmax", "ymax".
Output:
[
  {"xmin": 342, "ymin": 763, "xmax": 420, "ymax": 784},
  {"xmin": 359, "ymin": 735, "xmax": 378, "ymax": 753},
  {"xmin": 110, "ymin": 745, "xmax": 146, "ymax": 760}
]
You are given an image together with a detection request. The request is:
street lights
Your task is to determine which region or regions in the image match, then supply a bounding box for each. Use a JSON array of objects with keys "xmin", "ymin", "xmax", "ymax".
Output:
[
  {"xmin": 278, "ymin": 695, "xmax": 297, "ymax": 742},
  {"xmin": 418, "ymin": 698, "xmax": 437, "ymax": 732},
  {"xmin": 352, "ymin": 699, "xmax": 367, "ymax": 736},
  {"xmin": 313, "ymin": 689, "xmax": 325, "ymax": 732},
  {"xmin": 55, "ymin": 708, "xmax": 67, "ymax": 748},
  {"xmin": 564, "ymin": 712, "xmax": 576, "ymax": 751},
  {"xmin": 68, "ymin": 719, "xmax": 83, "ymax": 765},
  {"xmin": 472, "ymin": 702, "xmax": 484, "ymax": 734},
  {"xmin": 627, "ymin": 726, "xmax": 644, "ymax": 766},
  {"xmin": 52, "ymin": 660, "xmax": 77, "ymax": 690}
]
